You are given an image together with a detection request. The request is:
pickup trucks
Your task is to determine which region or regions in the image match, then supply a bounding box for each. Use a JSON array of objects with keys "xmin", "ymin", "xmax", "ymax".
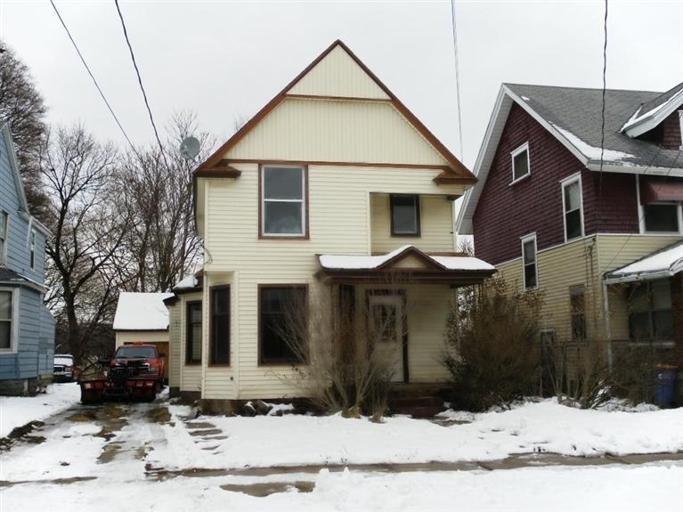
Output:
[{"xmin": 107, "ymin": 341, "xmax": 165, "ymax": 387}]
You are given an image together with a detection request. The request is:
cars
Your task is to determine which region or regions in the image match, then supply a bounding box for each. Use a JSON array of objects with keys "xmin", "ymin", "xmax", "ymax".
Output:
[{"xmin": 51, "ymin": 353, "xmax": 81, "ymax": 380}]
